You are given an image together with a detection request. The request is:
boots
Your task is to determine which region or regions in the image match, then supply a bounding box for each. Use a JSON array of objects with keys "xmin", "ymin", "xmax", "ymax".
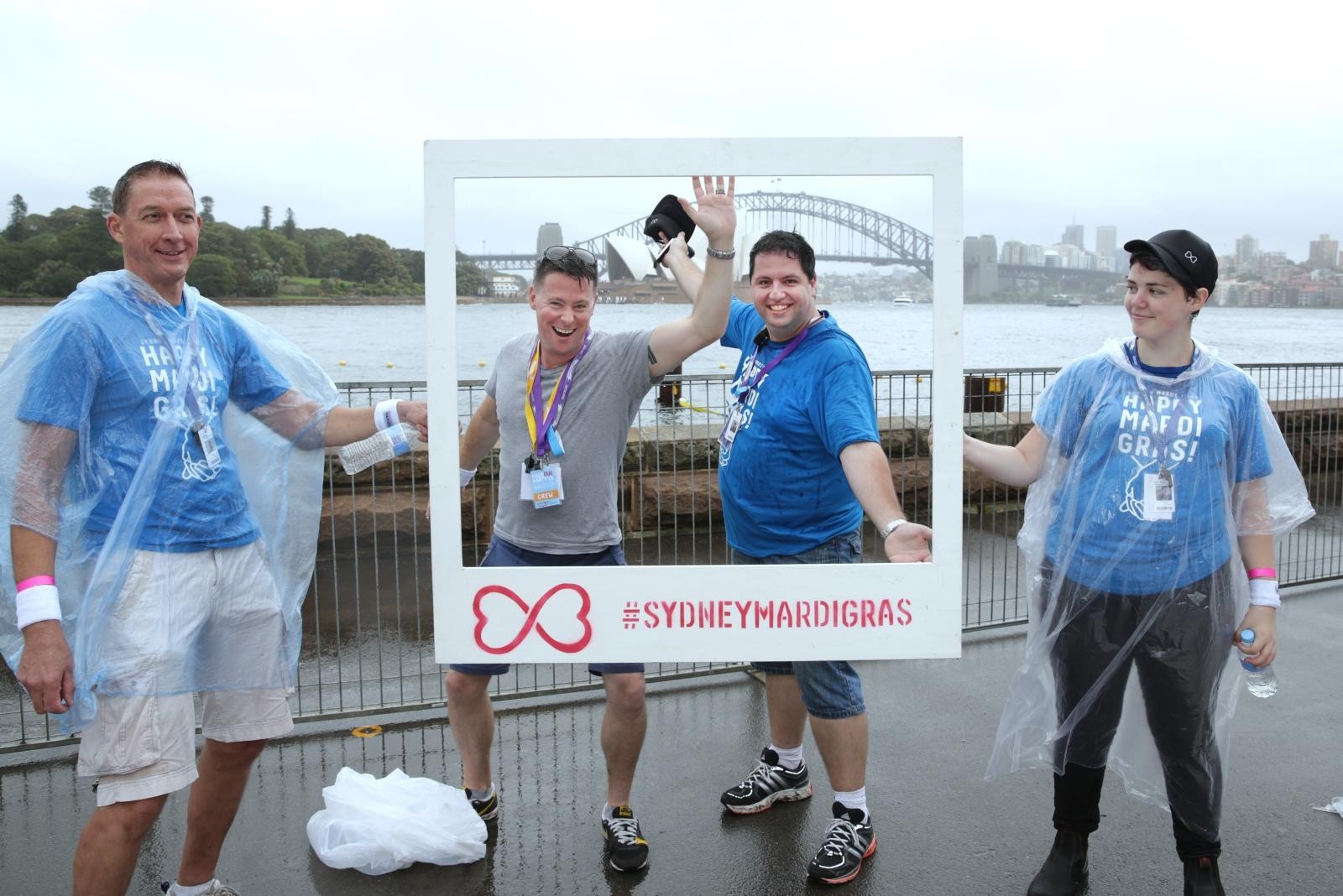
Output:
[
  {"xmin": 1184, "ymin": 856, "xmax": 1225, "ymax": 896},
  {"xmin": 1027, "ymin": 826, "xmax": 1090, "ymax": 896}
]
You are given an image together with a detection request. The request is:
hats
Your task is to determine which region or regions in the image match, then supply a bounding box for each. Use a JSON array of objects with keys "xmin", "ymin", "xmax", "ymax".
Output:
[
  {"xmin": 1123, "ymin": 229, "xmax": 1218, "ymax": 295},
  {"xmin": 644, "ymin": 195, "xmax": 696, "ymax": 258}
]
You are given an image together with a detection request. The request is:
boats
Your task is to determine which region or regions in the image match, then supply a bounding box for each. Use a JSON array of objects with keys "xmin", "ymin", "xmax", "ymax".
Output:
[
  {"xmin": 1046, "ymin": 293, "xmax": 1081, "ymax": 307},
  {"xmin": 893, "ymin": 294, "xmax": 913, "ymax": 307}
]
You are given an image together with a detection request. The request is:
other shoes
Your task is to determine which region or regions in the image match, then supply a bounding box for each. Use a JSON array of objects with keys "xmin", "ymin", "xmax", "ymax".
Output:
[{"xmin": 161, "ymin": 879, "xmax": 240, "ymax": 896}]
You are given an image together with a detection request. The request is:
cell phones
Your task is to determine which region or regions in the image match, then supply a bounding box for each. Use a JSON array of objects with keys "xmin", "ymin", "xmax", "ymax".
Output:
[{"xmin": 654, "ymin": 238, "xmax": 674, "ymax": 269}]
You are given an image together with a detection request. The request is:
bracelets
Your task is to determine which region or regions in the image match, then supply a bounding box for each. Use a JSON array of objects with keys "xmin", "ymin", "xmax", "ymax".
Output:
[
  {"xmin": 16, "ymin": 575, "xmax": 54, "ymax": 593},
  {"xmin": 707, "ymin": 247, "xmax": 736, "ymax": 259},
  {"xmin": 1247, "ymin": 579, "xmax": 1280, "ymax": 608},
  {"xmin": 1247, "ymin": 568, "xmax": 1276, "ymax": 581},
  {"xmin": 459, "ymin": 467, "xmax": 477, "ymax": 488},
  {"xmin": 881, "ymin": 519, "xmax": 907, "ymax": 543},
  {"xmin": 374, "ymin": 398, "xmax": 404, "ymax": 432},
  {"xmin": 15, "ymin": 584, "xmax": 62, "ymax": 631}
]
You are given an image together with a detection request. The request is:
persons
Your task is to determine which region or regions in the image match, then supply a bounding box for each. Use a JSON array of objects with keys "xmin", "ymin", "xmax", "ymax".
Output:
[
  {"xmin": 425, "ymin": 176, "xmax": 737, "ymax": 871},
  {"xmin": 10, "ymin": 160, "xmax": 463, "ymax": 895},
  {"xmin": 927, "ymin": 231, "xmax": 1279, "ymax": 896},
  {"xmin": 658, "ymin": 231, "xmax": 933, "ymax": 883}
]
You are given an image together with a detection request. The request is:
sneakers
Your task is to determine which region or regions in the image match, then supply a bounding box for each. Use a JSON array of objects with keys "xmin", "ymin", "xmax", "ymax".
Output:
[
  {"xmin": 807, "ymin": 802, "xmax": 875, "ymax": 883},
  {"xmin": 462, "ymin": 784, "xmax": 498, "ymax": 820},
  {"xmin": 720, "ymin": 746, "xmax": 812, "ymax": 813},
  {"xmin": 600, "ymin": 800, "xmax": 649, "ymax": 870}
]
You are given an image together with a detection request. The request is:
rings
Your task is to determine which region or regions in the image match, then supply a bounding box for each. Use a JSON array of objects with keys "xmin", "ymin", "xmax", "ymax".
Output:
[{"xmin": 715, "ymin": 189, "xmax": 725, "ymax": 194}]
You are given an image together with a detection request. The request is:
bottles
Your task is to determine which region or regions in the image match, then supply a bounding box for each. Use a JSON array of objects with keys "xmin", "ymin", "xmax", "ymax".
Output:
[
  {"xmin": 1237, "ymin": 630, "xmax": 1278, "ymax": 698},
  {"xmin": 338, "ymin": 422, "xmax": 422, "ymax": 476}
]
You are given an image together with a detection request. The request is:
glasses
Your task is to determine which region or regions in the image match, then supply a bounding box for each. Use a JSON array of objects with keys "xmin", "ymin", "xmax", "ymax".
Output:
[{"xmin": 545, "ymin": 244, "xmax": 598, "ymax": 271}]
